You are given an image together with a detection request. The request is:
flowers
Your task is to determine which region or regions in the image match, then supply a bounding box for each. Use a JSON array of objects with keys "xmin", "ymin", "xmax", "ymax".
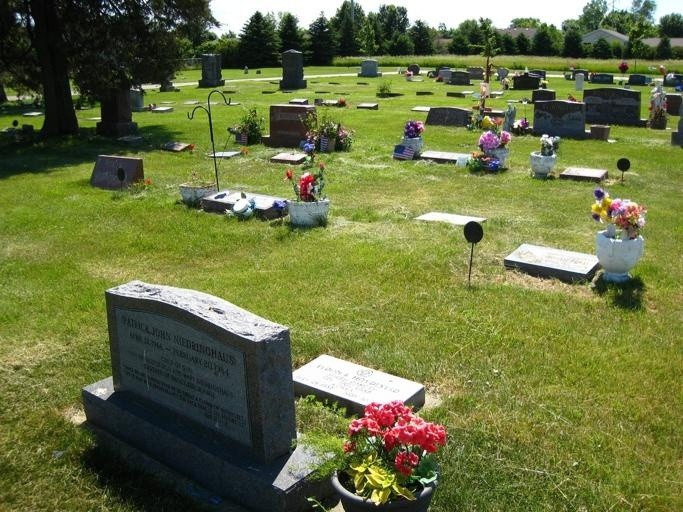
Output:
[
  {"xmin": 340, "ymin": 399, "xmax": 454, "ymax": 505},
  {"xmin": 586, "ymin": 189, "xmax": 647, "ymax": 236},
  {"xmin": 521, "ymin": 117, "xmax": 528, "ymax": 128},
  {"xmin": 538, "ymin": 133, "xmax": 561, "ymax": 154},
  {"xmin": 468, "ymin": 114, "xmax": 513, "ymax": 172},
  {"xmin": 567, "ymin": 92, "xmax": 582, "ymax": 103},
  {"xmin": 300, "ymin": 108, "xmax": 354, "ymax": 155},
  {"xmin": 649, "ymin": 87, "xmax": 668, "ymax": 121},
  {"xmin": 472, "ymin": 88, "xmax": 518, "ymax": 113},
  {"xmin": 403, "ymin": 118, "xmax": 426, "ymax": 136},
  {"xmin": 618, "ymin": 63, "xmax": 629, "ymax": 69},
  {"xmin": 286, "ymin": 162, "xmax": 329, "ymax": 203},
  {"xmin": 337, "ymin": 97, "xmax": 350, "ymax": 108}
]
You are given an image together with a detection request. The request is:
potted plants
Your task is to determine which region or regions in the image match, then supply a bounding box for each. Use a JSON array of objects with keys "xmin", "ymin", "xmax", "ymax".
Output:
[
  {"xmin": 177, "ymin": 169, "xmax": 217, "ymax": 207},
  {"xmin": 227, "ymin": 106, "xmax": 265, "ymax": 146}
]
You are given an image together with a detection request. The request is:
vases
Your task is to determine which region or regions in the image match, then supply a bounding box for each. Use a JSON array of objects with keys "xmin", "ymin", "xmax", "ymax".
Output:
[
  {"xmin": 620, "ymin": 68, "xmax": 627, "ymax": 73},
  {"xmin": 594, "ymin": 230, "xmax": 643, "ymax": 283},
  {"xmin": 328, "ymin": 466, "xmax": 441, "ymax": 512}
]
